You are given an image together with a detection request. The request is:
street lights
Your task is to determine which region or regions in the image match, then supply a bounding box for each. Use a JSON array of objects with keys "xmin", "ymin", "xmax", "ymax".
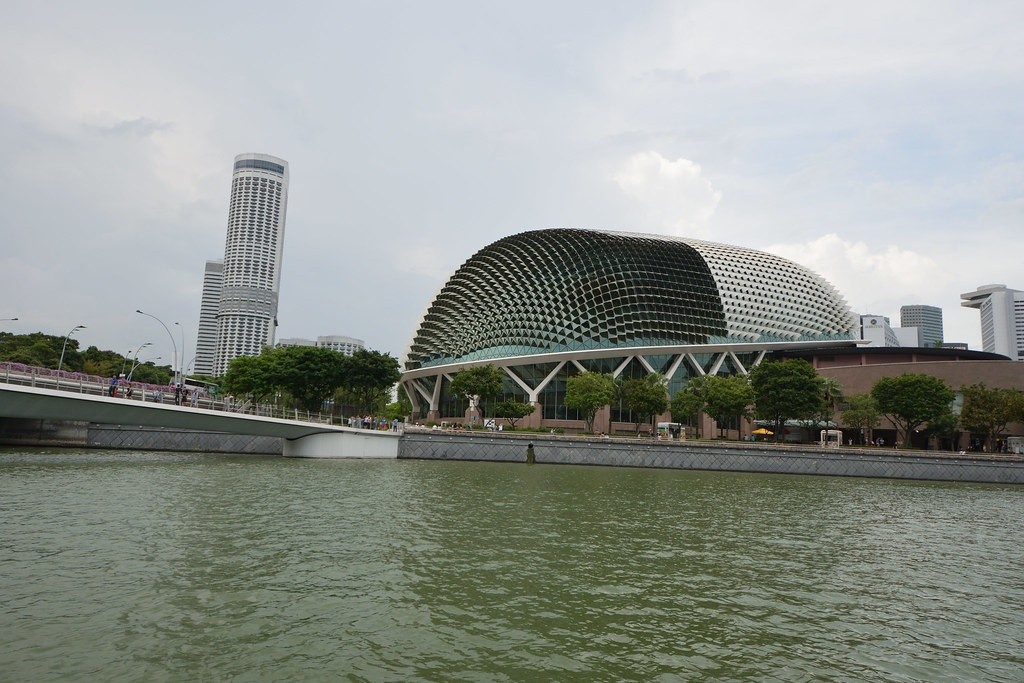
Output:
[
  {"xmin": 57, "ymin": 326, "xmax": 87, "ymax": 390},
  {"xmin": 182, "ymin": 356, "xmax": 203, "ymax": 388},
  {"xmin": 129, "ymin": 343, "xmax": 151, "ymax": 382},
  {"xmin": 136, "ymin": 310, "xmax": 177, "ymax": 388},
  {"xmin": 119, "ymin": 350, "xmax": 131, "ymax": 399},
  {"xmin": 126, "ymin": 357, "xmax": 162, "ymax": 382},
  {"xmin": 175, "ymin": 323, "xmax": 183, "ymax": 405}
]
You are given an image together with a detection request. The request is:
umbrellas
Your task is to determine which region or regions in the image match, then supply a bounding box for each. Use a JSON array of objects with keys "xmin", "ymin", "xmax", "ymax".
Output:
[{"xmin": 751, "ymin": 428, "xmax": 773, "ymax": 435}]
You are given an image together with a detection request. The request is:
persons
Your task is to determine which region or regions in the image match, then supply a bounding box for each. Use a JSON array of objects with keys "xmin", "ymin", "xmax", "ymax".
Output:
[
  {"xmin": 152, "ymin": 386, "xmax": 159, "ymax": 403},
  {"xmin": 959, "ymin": 445, "xmax": 963, "ymax": 450},
  {"xmin": 432, "ymin": 424, "xmax": 442, "ymax": 430},
  {"xmin": 109, "ymin": 376, "xmax": 118, "ymax": 397},
  {"xmin": 893, "ymin": 442, "xmax": 898, "ymax": 449},
  {"xmin": 347, "ymin": 414, "xmax": 398, "ymax": 433},
  {"xmin": 743, "ymin": 434, "xmax": 748, "ymax": 441},
  {"xmin": 222, "ymin": 395, "xmax": 235, "ymax": 412},
  {"xmin": 848, "ymin": 438, "xmax": 853, "ymax": 445},
  {"xmin": 595, "ymin": 429, "xmax": 605, "ymax": 437},
  {"xmin": 751, "ymin": 435, "xmax": 755, "ymax": 441},
  {"xmin": 125, "ymin": 383, "xmax": 132, "ymax": 399},
  {"xmin": 453, "ymin": 422, "xmax": 463, "ymax": 430},
  {"xmin": 870, "ymin": 437, "xmax": 884, "ymax": 447},
  {"xmin": 488, "ymin": 423, "xmax": 502, "ymax": 432},
  {"xmin": 968, "ymin": 443, "xmax": 973, "ymax": 451},
  {"xmin": 1001, "ymin": 442, "xmax": 1011, "ymax": 453},
  {"xmin": 982, "ymin": 444, "xmax": 987, "ymax": 452},
  {"xmin": 175, "ymin": 384, "xmax": 199, "ymax": 407},
  {"xmin": 248, "ymin": 394, "xmax": 256, "ymax": 414},
  {"xmin": 415, "ymin": 422, "xmax": 425, "ymax": 428}
]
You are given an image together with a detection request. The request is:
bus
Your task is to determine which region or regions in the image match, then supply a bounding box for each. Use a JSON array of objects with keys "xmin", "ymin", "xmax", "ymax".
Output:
[{"xmin": 169, "ymin": 377, "xmax": 218, "ymax": 398}]
